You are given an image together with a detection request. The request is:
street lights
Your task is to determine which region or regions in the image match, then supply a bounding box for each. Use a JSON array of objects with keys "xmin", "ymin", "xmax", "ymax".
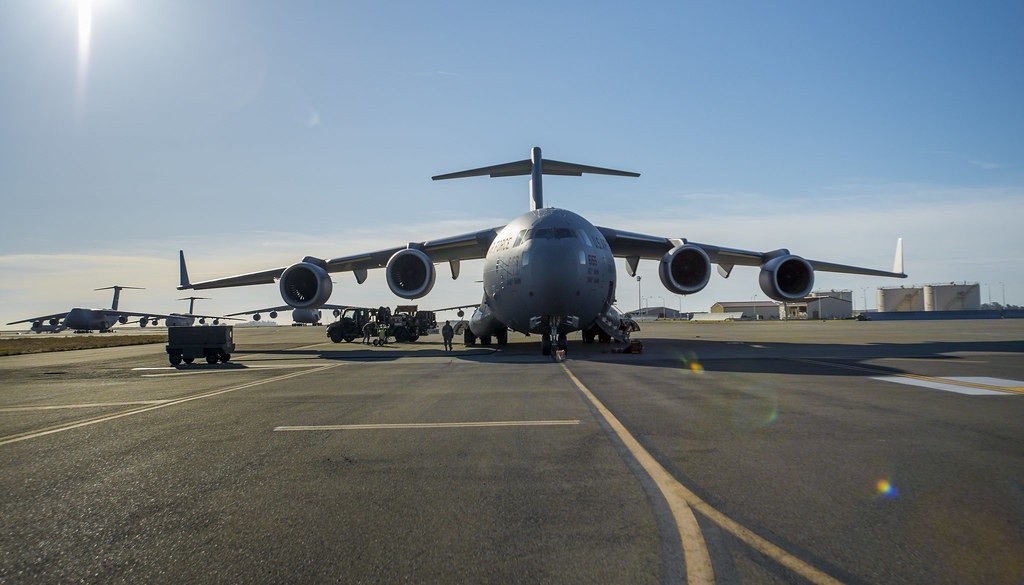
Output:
[
  {"xmin": 674, "ymin": 295, "xmax": 681, "ymax": 319},
  {"xmin": 754, "ymin": 295, "xmax": 757, "ymax": 314},
  {"xmin": 658, "ymin": 296, "xmax": 665, "ymax": 318},
  {"xmin": 861, "ymin": 287, "xmax": 869, "ymax": 309}
]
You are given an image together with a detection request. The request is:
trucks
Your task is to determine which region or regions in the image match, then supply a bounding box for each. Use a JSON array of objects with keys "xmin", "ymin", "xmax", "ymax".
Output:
[{"xmin": 326, "ymin": 305, "xmax": 435, "ymax": 342}]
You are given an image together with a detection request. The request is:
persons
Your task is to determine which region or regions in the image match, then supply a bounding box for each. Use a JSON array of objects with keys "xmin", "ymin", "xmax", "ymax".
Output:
[
  {"xmin": 441, "ymin": 321, "xmax": 453, "ymax": 351},
  {"xmin": 361, "ymin": 321, "xmax": 375, "ymax": 345}
]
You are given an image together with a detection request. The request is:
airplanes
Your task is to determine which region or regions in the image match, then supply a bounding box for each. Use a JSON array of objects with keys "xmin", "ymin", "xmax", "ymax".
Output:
[
  {"xmin": 6, "ymin": 286, "xmax": 188, "ymax": 334},
  {"xmin": 177, "ymin": 146, "xmax": 908, "ymax": 362},
  {"xmin": 120, "ymin": 297, "xmax": 247, "ymax": 327},
  {"xmin": 224, "ymin": 304, "xmax": 356, "ymax": 326}
]
